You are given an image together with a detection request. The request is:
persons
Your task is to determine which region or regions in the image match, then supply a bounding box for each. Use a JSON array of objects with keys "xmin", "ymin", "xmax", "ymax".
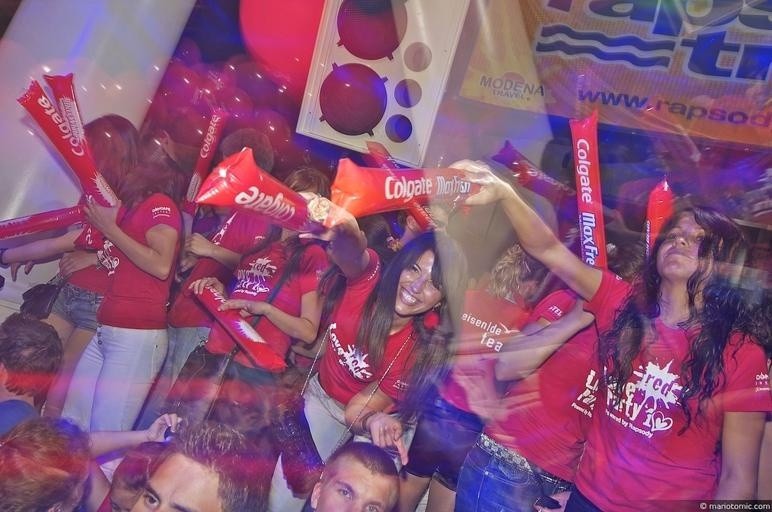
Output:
[{"xmin": 0, "ymin": 113, "xmax": 772, "ymax": 512}]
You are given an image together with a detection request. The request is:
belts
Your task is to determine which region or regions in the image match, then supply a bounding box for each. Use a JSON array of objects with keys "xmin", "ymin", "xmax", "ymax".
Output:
[
  {"xmin": 476, "ymin": 432, "xmax": 561, "ymax": 485},
  {"xmin": 427, "ymin": 398, "xmax": 457, "ymax": 412}
]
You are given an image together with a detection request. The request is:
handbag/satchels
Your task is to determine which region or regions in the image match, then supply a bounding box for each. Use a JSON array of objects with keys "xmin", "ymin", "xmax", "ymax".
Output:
[
  {"xmin": 268, "ymin": 394, "xmax": 324, "ymax": 499},
  {"xmin": 160, "ymin": 344, "xmax": 230, "ymax": 424},
  {"xmin": 19, "ymin": 285, "xmax": 59, "ymax": 319}
]
